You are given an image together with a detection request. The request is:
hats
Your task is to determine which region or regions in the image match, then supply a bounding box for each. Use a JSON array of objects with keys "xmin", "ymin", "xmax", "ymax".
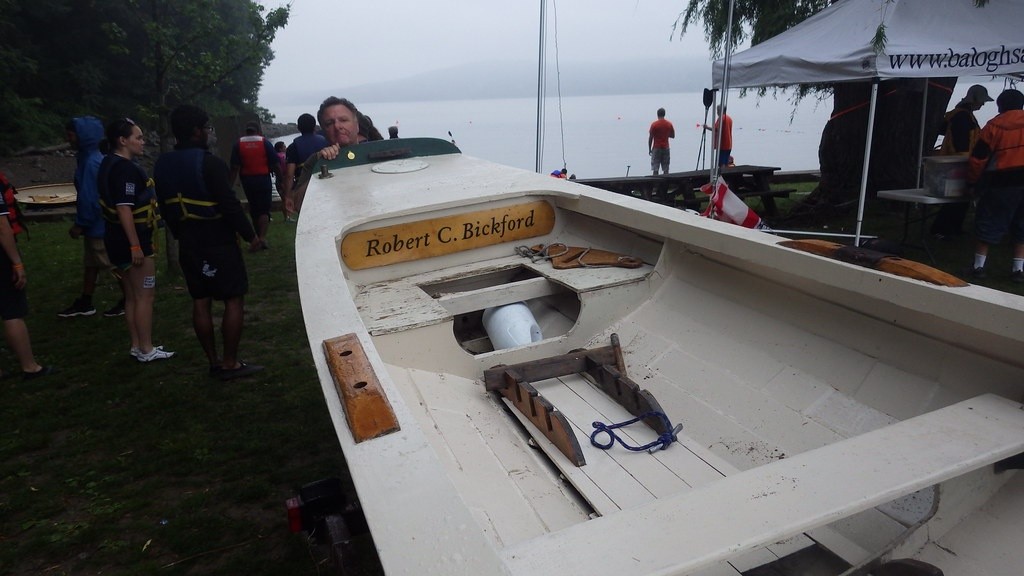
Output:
[{"xmin": 971, "ymin": 84, "xmax": 993, "ymax": 100}]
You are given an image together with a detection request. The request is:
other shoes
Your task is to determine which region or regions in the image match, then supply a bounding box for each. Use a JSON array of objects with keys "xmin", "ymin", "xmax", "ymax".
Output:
[
  {"xmin": 260, "ymin": 241, "xmax": 270, "ymax": 250},
  {"xmin": 208, "ymin": 364, "xmax": 220, "ymax": 376},
  {"xmin": 963, "ymin": 266, "xmax": 985, "ymax": 283},
  {"xmin": 220, "ymin": 362, "xmax": 266, "ymax": 380},
  {"xmin": 1011, "ymin": 269, "xmax": 1024, "ymax": 283},
  {"xmin": 23, "ymin": 366, "xmax": 60, "ymax": 379}
]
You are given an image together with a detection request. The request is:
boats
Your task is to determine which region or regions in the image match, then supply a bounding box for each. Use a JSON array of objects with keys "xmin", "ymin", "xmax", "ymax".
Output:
[
  {"xmin": 284, "ymin": 123, "xmax": 1023, "ymax": 576},
  {"xmin": 14, "ymin": 182, "xmax": 78, "ymax": 206}
]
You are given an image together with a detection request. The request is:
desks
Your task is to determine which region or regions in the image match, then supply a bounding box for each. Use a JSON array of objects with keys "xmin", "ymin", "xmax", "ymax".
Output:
[
  {"xmin": 878, "ymin": 189, "xmax": 965, "ymax": 268},
  {"xmin": 567, "ymin": 164, "xmax": 784, "ymax": 201}
]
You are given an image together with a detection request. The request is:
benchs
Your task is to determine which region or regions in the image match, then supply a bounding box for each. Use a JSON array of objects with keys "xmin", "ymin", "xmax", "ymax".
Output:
[
  {"xmin": 508, "ymin": 389, "xmax": 1024, "ymax": 576},
  {"xmin": 646, "ymin": 185, "xmax": 798, "ymax": 206}
]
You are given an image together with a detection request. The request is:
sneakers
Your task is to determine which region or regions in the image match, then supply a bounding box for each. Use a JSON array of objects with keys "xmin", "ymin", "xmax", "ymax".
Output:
[
  {"xmin": 56, "ymin": 297, "xmax": 96, "ymax": 317},
  {"xmin": 104, "ymin": 297, "xmax": 127, "ymax": 316},
  {"xmin": 130, "ymin": 343, "xmax": 178, "ymax": 363}
]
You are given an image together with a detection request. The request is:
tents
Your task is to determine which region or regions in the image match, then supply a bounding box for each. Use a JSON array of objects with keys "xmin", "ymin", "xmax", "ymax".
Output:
[{"xmin": 713, "ymin": 0, "xmax": 1024, "ymax": 252}]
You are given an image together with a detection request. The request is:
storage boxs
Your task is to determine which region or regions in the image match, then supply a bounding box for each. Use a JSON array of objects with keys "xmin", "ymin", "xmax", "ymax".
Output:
[{"xmin": 921, "ymin": 157, "xmax": 971, "ymax": 196}]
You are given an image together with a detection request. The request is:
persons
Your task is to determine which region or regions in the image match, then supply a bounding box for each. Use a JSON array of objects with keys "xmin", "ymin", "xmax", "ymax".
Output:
[
  {"xmin": 550, "ymin": 167, "xmax": 568, "ymax": 180},
  {"xmin": 648, "ymin": 108, "xmax": 674, "ymax": 176},
  {"xmin": 154, "ymin": 106, "xmax": 265, "ymax": 377},
  {"xmin": 933, "ymin": 84, "xmax": 1024, "ymax": 290},
  {"xmin": 228, "ymin": 95, "xmax": 386, "ymax": 251},
  {"xmin": 703, "ymin": 104, "xmax": 732, "ymax": 170},
  {"xmin": 0, "ymin": 167, "xmax": 49, "ymax": 378},
  {"xmin": 99, "ymin": 117, "xmax": 176, "ymax": 364},
  {"xmin": 54, "ymin": 116, "xmax": 127, "ymax": 317}
]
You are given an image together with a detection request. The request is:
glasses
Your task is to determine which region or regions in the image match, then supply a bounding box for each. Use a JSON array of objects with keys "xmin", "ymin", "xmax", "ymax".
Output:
[{"xmin": 123, "ymin": 118, "xmax": 135, "ymax": 127}]
